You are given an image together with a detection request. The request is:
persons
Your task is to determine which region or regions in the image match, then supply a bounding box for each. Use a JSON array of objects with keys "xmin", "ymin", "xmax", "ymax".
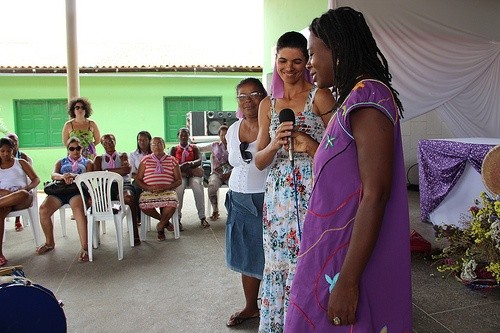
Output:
[
  {"xmin": 0, "ymin": 138, "xmax": 40, "ymax": 266},
  {"xmin": 128, "ymin": 131, "xmax": 151, "ymax": 224},
  {"xmin": 38, "ymin": 137, "xmax": 94, "ymax": 263},
  {"xmin": 170, "ymin": 128, "xmax": 210, "ymax": 231},
  {"xmin": 254, "ymin": 32, "xmax": 337, "ymax": 333},
  {"xmin": 285, "ymin": 6, "xmax": 412, "ymax": 333},
  {"xmin": 88, "ymin": 134, "xmax": 141, "ymax": 246},
  {"xmin": 226, "ymin": 78, "xmax": 268, "ymax": 326},
  {"xmin": 136, "ymin": 137, "xmax": 182, "ymax": 242},
  {"xmin": 62, "ymin": 96, "xmax": 100, "ymax": 162},
  {"xmin": 190, "ymin": 125, "xmax": 233, "ymax": 221},
  {"xmin": 7, "ymin": 133, "xmax": 40, "ymax": 231}
]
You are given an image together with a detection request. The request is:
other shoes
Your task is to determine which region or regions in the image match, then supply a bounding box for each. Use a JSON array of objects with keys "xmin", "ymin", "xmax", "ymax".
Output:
[
  {"xmin": 15, "ymin": 222, "xmax": 23, "ymax": 231},
  {"xmin": 201, "ymin": 219, "xmax": 210, "ymax": 227},
  {"xmin": 211, "ymin": 211, "xmax": 218, "ymax": 221},
  {"xmin": 180, "ymin": 224, "xmax": 184, "ymax": 231},
  {"xmin": 0, "ymin": 256, "xmax": 8, "ymax": 265},
  {"xmin": 156, "ymin": 222, "xmax": 165, "ymax": 240},
  {"xmin": 137, "ymin": 218, "xmax": 141, "ymax": 225},
  {"xmin": 71, "ymin": 215, "xmax": 75, "ymax": 220},
  {"xmin": 164, "ymin": 223, "xmax": 174, "ymax": 231},
  {"xmin": 134, "ymin": 234, "xmax": 140, "ymax": 246}
]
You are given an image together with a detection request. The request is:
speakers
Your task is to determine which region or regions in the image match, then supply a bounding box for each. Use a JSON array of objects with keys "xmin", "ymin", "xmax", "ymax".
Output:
[{"xmin": 185, "ymin": 111, "xmax": 240, "ymax": 137}]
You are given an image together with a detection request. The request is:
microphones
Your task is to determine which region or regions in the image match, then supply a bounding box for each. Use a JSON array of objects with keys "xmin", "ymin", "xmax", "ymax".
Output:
[{"xmin": 278, "ymin": 107, "xmax": 295, "ymax": 167}]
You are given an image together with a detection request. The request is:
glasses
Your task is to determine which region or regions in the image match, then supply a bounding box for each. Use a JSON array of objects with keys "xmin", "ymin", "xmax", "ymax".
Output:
[
  {"xmin": 69, "ymin": 146, "xmax": 82, "ymax": 151},
  {"xmin": 74, "ymin": 106, "xmax": 85, "ymax": 110},
  {"xmin": 237, "ymin": 92, "xmax": 263, "ymax": 100},
  {"xmin": 240, "ymin": 141, "xmax": 253, "ymax": 164}
]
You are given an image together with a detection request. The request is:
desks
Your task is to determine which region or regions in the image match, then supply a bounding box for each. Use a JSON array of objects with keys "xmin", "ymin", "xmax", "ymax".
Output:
[{"xmin": 416, "ymin": 138, "xmax": 500, "ymax": 231}]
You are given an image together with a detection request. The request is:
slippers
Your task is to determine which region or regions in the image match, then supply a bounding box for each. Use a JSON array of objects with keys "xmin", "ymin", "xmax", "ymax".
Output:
[{"xmin": 226, "ymin": 312, "xmax": 260, "ymax": 327}]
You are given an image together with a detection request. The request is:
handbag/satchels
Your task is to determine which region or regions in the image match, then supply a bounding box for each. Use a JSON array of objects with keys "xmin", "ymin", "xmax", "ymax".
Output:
[
  {"xmin": 191, "ymin": 166, "xmax": 205, "ymax": 176},
  {"xmin": 89, "ymin": 142, "xmax": 96, "ymax": 157},
  {"xmin": 43, "ymin": 180, "xmax": 65, "ymax": 195},
  {"xmin": 214, "ymin": 162, "xmax": 234, "ymax": 182}
]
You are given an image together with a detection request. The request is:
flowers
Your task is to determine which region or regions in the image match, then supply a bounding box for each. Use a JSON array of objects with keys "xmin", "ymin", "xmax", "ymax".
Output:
[{"xmin": 431, "ymin": 192, "xmax": 500, "ymax": 285}]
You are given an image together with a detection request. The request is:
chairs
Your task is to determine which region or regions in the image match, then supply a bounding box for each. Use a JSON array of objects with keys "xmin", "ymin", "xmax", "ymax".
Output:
[
  {"xmin": 75, "ymin": 171, "xmax": 134, "ymax": 262},
  {"xmin": 50, "ymin": 203, "xmax": 74, "ymax": 238},
  {"xmin": 6, "ymin": 188, "xmax": 41, "ymax": 247},
  {"xmin": 141, "ymin": 207, "xmax": 180, "ymax": 241}
]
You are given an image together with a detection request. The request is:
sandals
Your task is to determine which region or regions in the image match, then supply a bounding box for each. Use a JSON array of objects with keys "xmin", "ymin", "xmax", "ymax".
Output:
[
  {"xmin": 79, "ymin": 249, "xmax": 88, "ymax": 263},
  {"xmin": 36, "ymin": 243, "xmax": 54, "ymax": 255}
]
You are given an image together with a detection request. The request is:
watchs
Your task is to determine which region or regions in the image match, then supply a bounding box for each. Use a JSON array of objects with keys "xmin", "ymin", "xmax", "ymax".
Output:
[{"xmin": 106, "ymin": 169, "xmax": 108, "ymax": 172}]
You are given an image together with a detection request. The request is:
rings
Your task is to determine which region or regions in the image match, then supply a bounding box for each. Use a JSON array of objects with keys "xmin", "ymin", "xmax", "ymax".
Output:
[{"xmin": 333, "ymin": 316, "xmax": 340, "ymax": 325}]
